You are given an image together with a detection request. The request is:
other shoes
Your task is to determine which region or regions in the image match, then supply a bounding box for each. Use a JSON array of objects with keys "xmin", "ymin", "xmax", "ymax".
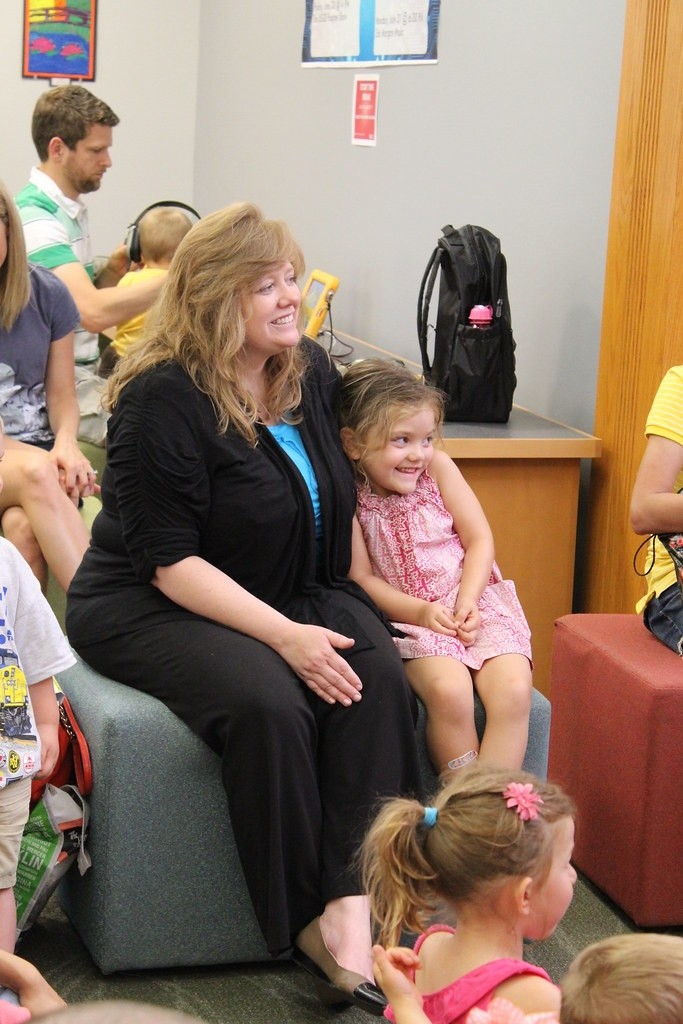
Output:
[{"xmin": 291, "ymin": 916, "xmax": 389, "ymax": 1008}]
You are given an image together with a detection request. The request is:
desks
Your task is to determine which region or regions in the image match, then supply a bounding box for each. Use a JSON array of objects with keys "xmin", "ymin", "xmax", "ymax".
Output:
[{"xmin": 304, "ymin": 325, "xmax": 602, "ymax": 694}]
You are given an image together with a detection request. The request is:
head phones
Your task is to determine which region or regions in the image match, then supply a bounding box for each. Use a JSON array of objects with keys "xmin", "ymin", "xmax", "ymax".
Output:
[{"xmin": 124, "ymin": 201, "xmax": 200, "ymax": 263}]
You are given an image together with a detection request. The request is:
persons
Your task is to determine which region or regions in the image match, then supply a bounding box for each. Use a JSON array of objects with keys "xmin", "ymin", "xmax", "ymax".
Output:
[
  {"xmin": 13, "ymin": 86, "xmax": 170, "ymax": 446},
  {"xmin": 372, "ymin": 933, "xmax": 683, "ymax": 1024},
  {"xmin": 342, "ymin": 358, "xmax": 532, "ymax": 778},
  {"xmin": 631, "ymin": 366, "xmax": 683, "ymax": 656},
  {"xmin": 363, "ymin": 762, "xmax": 577, "ymax": 1024},
  {"xmin": 0, "ymin": 178, "xmax": 101, "ymax": 595},
  {"xmin": 99, "ymin": 208, "xmax": 193, "ymax": 381},
  {"xmin": 66, "ymin": 203, "xmax": 428, "ymax": 1008},
  {"xmin": 0, "ymin": 419, "xmax": 76, "ymax": 952}
]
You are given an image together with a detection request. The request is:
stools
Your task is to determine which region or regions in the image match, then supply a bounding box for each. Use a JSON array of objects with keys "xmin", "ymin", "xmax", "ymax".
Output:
[
  {"xmin": 56, "ymin": 647, "xmax": 548, "ymax": 979},
  {"xmin": 548, "ymin": 610, "xmax": 683, "ymax": 933}
]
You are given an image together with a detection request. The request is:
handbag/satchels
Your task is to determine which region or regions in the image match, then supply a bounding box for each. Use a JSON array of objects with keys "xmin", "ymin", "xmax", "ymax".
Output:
[{"xmin": 13, "ymin": 697, "xmax": 92, "ymax": 943}]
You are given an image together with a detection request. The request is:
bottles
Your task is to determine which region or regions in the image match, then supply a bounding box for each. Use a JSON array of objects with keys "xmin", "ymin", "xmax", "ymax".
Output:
[{"xmin": 468, "ymin": 305, "xmax": 493, "ymax": 329}]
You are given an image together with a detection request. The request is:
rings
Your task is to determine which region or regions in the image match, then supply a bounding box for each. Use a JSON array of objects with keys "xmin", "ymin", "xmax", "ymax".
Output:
[{"xmin": 88, "ymin": 470, "xmax": 98, "ymax": 475}]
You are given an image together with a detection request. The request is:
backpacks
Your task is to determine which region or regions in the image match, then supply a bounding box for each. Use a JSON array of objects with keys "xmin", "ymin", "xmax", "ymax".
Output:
[{"xmin": 417, "ymin": 224, "xmax": 516, "ymax": 425}]
